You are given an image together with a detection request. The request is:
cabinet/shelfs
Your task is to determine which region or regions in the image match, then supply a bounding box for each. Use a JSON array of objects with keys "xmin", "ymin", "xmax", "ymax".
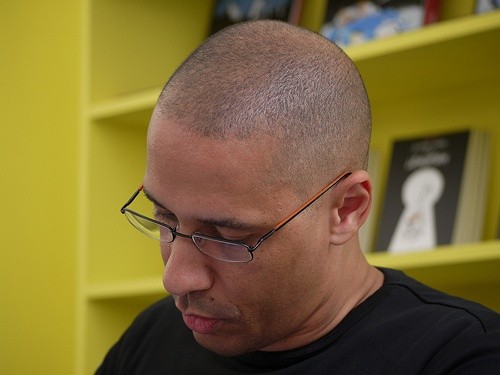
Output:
[{"xmin": 79, "ymin": 0, "xmax": 500, "ymax": 375}]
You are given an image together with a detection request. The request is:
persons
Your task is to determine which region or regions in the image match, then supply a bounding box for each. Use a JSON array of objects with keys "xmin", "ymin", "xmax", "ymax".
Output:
[{"xmin": 94, "ymin": 21, "xmax": 500, "ymax": 374}]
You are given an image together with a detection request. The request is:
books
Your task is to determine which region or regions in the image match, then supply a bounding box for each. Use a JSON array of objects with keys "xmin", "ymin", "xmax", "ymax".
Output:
[{"xmin": 373, "ymin": 125, "xmax": 491, "ymax": 256}]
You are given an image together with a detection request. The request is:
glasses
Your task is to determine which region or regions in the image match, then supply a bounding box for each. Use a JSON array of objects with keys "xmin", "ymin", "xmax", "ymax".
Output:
[{"xmin": 120, "ymin": 170, "xmax": 353, "ymax": 264}]
